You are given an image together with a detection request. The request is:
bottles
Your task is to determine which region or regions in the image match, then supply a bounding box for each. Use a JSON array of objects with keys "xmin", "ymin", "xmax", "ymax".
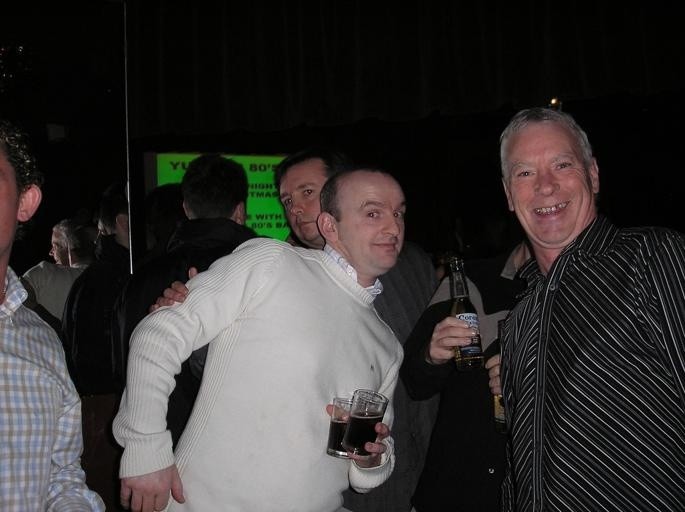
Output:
[
  {"xmin": 491, "ymin": 318, "xmax": 509, "ymax": 425},
  {"xmin": 446, "ymin": 254, "xmax": 489, "ymax": 374}
]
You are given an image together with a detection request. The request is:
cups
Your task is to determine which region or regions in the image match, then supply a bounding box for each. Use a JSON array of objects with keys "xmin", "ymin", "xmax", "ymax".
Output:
[
  {"xmin": 341, "ymin": 387, "xmax": 390, "ymax": 456},
  {"xmin": 325, "ymin": 395, "xmax": 361, "ymax": 461}
]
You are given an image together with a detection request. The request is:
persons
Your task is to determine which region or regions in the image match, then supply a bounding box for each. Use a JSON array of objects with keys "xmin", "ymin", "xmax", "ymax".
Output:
[
  {"xmin": 148, "ymin": 144, "xmax": 440, "ymax": 511},
  {"xmin": 22, "ymin": 153, "xmax": 256, "ymax": 511},
  {"xmin": 401, "ymin": 239, "xmax": 535, "ymax": 511},
  {"xmin": 494, "ymin": 104, "xmax": 684, "ymax": 512},
  {"xmin": 0, "ymin": 120, "xmax": 112, "ymax": 511},
  {"xmin": 111, "ymin": 164, "xmax": 407, "ymax": 512}
]
